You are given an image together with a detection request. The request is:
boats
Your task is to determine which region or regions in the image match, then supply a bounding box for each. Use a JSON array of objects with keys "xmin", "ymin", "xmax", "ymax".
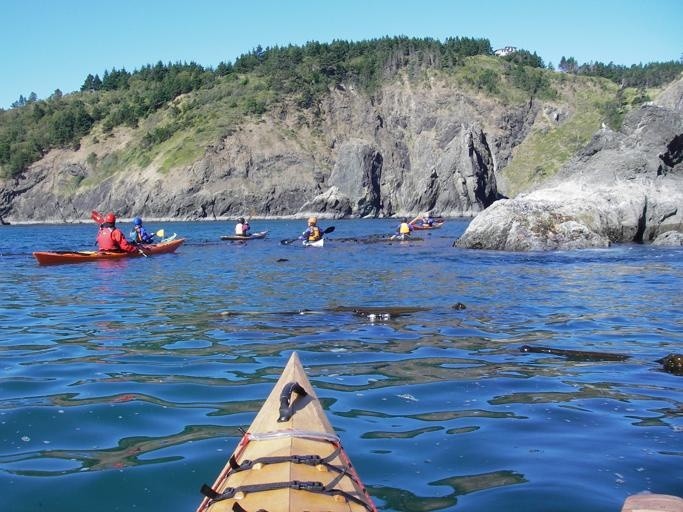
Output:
[
  {"xmin": 405, "ymin": 221, "xmax": 446, "ymax": 230},
  {"xmin": 398, "ymin": 234, "xmax": 413, "ymax": 240},
  {"xmin": 219, "ymin": 230, "xmax": 269, "ymax": 241},
  {"xmin": 191, "ymin": 349, "xmax": 378, "ymax": 512},
  {"xmin": 32, "ymin": 233, "xmax": 187, "ymax": 266},
  {"xmin": 303, "ymin": 238, "xmax": 326, "ymax": 248}
]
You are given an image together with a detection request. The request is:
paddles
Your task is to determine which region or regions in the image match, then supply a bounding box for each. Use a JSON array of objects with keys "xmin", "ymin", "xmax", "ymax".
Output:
[
  {"xmin": 91, "ymin": 211, "xmax": 148, "ymax": 258},
  {"xmin": 246, "ymin": 210, "xmax": 255, "ymax": 224},
  {"xmin": 390, "ymin": 214, "xmax": 423, "ymax": 241},
  {"xmin": 154, "ymin": 229, "xmax": 164, "ymax": 237},
  {"xmin": 281, "ymin": 227, "xmax": 335, "ymax": 245}
]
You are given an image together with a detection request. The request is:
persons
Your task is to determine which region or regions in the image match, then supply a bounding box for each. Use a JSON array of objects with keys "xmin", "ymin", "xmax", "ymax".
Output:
[
  {"xmin": 129, "ymin": 217, "xmax": 153, "ymax": 244},
  {"xmin": 95, "ymin": 212, "xmax": 145, "ymax": 256},
  {"xmin": 297, "ymin": 216, "xmax": 324, "ymax": 243},
  {"xmin": 394, "ymin": 216, "xmax": 414, "ymax": 238},
  {"xmin": 418, "ymin": 216, "xmax": 427, "ymax": 226},
  {"xmin": 233, "ymin": 218, "xmax": 253, "ymax": 238},
  {"xmin": 426, "ymin": 216, "xmax": 434, "ymax": 227}
]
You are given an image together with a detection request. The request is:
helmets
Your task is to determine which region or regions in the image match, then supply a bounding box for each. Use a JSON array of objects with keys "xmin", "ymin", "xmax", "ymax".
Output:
[
  {"xmin": 105, "ymin": 213, "xmax": 115, "ymax": 223},
  {"xmin": 305, "ymin": 216, "xmax": 317, "ymax": 224},
  {"xmin": 399, "ymin": 217, "xmax": 408, "ymax": 223},
  {"xmin": 133, "ymin": 217, "xmax": 142, "ymax": 225},
  {"xmin": 238, "ymin": 217, "xmax": 245, "ymax": 223}
]
